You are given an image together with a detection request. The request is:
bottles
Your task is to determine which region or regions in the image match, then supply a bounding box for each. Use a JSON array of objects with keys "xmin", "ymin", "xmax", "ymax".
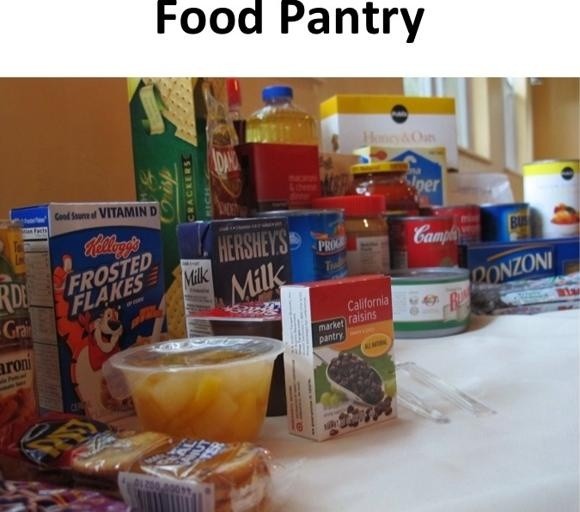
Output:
[
  {"xmin": 226, "ymin": 80, "xmax": 247, "ymax": 133},
  {"xmin": 245, "ymin": 84, "xmax": 320, "ymax": 209}
]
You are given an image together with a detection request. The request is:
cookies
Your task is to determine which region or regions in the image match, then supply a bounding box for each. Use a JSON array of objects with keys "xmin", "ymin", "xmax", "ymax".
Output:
[
  {"xmin": 140, "ymin": 78, "xmax": 198, "ymax": 148},
  {"xmin": 70, "ymin": 422, "xmax": 273, "ymax": 512}
]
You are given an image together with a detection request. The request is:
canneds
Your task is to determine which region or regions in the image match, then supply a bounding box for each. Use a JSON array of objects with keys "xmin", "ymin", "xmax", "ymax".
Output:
[{"xmin": 256, "ymin": 159, "xmax": 580, "ymax": 341}]
